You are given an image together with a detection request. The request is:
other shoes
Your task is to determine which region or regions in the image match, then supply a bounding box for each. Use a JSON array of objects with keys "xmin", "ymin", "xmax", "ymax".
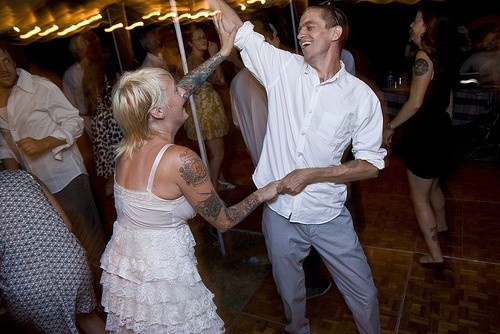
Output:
[
  {"xmin": 306, "ymin": 278, "xmax": 332, "ymax": 301},
  {"xmin": 412, "ymin": 253, "xmax": 446, "ymax": 269},
  {"xmin": 217, "ymin": 180, "xmax": 238, "ymax": 192}
]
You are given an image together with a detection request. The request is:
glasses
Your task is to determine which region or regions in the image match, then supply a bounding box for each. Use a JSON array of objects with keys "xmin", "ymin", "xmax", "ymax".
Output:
[
  {"xmin": 318, "ymin": 1, "xmax": 339, "ymax": 24},
  {"xmin": 190, "ymin": 35, "xmax": 209, "ymax": 41}
]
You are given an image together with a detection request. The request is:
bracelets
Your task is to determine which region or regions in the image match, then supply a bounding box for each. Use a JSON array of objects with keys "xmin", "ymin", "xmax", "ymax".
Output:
[{"xmin": 407, "ymin": 41, "xmax": 411, "ymax": 45}]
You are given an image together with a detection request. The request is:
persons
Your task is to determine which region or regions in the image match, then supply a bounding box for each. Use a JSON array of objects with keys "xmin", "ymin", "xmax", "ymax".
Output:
[
  {"xmin": 340, "ymin": 49, "xmax": 364, "ymax": 231},
  {"xmin": 0, "ymin": 45, "xmax": 106, "ymax": 313},
  {"xmin": 208, "ymin": 0, "xmax": 388, "ymax": 334},
  {"xmin": 99, "ymin": 13, "xmax": 282, "ymax": 334},
  {"xmin": 383, "ymin": 8, "xmax": 451, "ymax": 271},
  {"xmin": 229, "ymin": 20, "xmax": 331, "ymax": 303},
  {"xmin": 0, "ymin": 167, "xmax": 108, "ymax": 334},
  {"xmin": 63, "ymin": 1, "xmax": 236, "ymax": 197},
  {"xmin": 405, "ymin": 24, "xmax": 500, "ymax": 200}
]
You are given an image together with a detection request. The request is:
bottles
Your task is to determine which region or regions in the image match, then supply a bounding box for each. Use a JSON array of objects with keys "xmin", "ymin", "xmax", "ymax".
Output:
[{"xmin": 387, "ymin": 71, "xmax": 394, "ymax": 87}]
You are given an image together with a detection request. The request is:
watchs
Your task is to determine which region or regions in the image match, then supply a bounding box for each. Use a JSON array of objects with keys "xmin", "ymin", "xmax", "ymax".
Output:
[{"xmin": 387, "ymin": 121, "xmax": 393, "ymax": 132}]
